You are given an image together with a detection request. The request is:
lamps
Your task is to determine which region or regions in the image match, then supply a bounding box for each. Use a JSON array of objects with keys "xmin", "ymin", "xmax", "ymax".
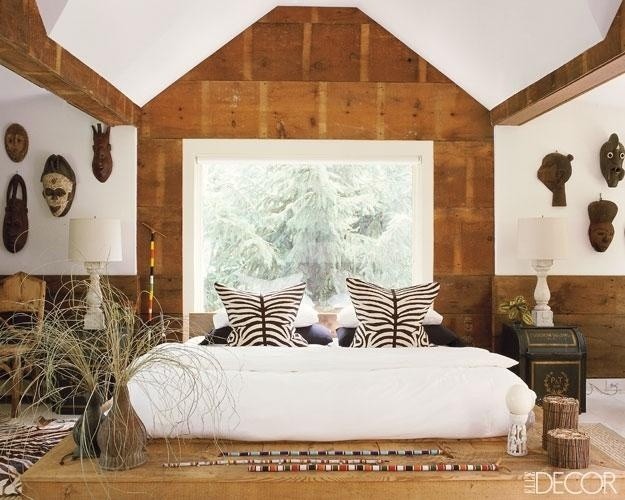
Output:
[
  {"xmin": 66, "ymin": 215, "xmax": 124, "ymax": 329},
  {"xmin": 514, "ymin": 215, "xmax": 572, "ymax": 327}
]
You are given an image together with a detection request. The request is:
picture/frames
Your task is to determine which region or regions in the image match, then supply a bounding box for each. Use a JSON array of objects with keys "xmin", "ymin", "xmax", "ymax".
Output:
[{"xmin": 179, "ymin": 139, "xmax": 438, "ymax": 339}]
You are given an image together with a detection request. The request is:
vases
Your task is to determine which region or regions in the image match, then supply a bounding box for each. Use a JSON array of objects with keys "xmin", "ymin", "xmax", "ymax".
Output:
[{"xmin": 73, "ymin": 381, "xmax": 149, "ymax": 472}]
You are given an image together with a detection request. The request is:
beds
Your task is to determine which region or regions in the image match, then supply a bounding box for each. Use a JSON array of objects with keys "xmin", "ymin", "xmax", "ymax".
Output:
[{"xmin": 109, "ymin": 337, "xmax": 538, "ymax": 440}]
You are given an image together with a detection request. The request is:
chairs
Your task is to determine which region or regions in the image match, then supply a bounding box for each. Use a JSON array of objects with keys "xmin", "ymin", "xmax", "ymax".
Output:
[{"xmin": 1, "ymin": 272, "xmax": 44, "ymax": 420}]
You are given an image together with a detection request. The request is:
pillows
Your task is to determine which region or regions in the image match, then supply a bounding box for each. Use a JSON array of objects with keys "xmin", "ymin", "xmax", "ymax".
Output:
[{"xmin": 200, "ymin": 277, "xmax": 462, "ymax": 347}]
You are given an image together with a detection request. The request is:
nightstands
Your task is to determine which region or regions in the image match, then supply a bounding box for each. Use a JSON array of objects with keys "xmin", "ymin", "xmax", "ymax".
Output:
[
  {"xmin": 505, "ymin": 323, "xmax": 588, "ymax": 412},
  {"xmin": 51, "ymin": 326, "xmax": 171, "ymax": 414}
]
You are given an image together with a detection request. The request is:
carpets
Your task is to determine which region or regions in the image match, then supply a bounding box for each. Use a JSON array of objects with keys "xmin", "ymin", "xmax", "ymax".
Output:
[{"xmin": 0, "ymin": 420, "xmax": 78, "ymax": 499}]
